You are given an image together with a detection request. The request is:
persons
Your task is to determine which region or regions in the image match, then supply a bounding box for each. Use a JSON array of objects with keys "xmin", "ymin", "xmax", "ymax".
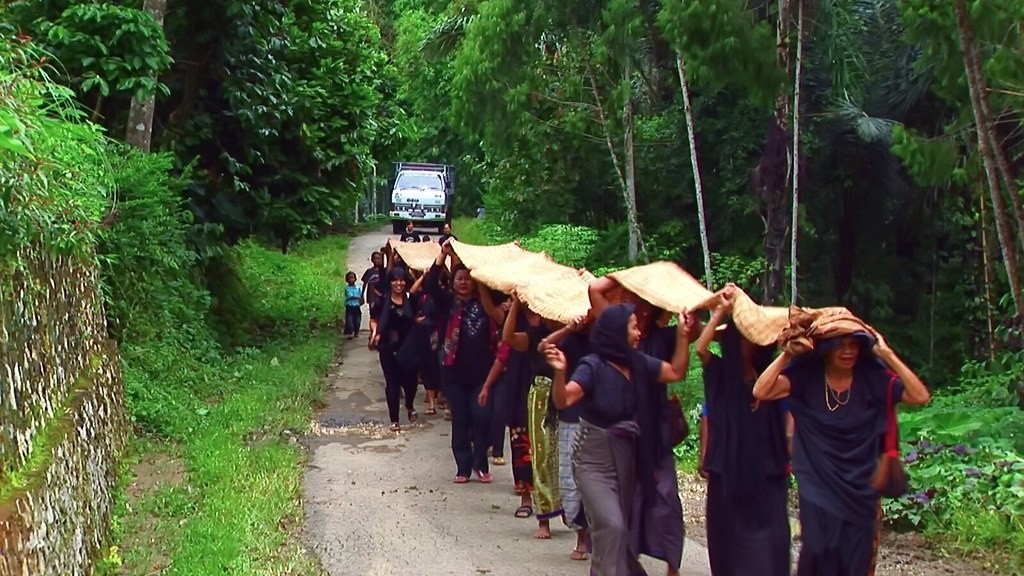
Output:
[{"xmin": 343, "ymin": 220, "xmax": 930, "ymax": 576}]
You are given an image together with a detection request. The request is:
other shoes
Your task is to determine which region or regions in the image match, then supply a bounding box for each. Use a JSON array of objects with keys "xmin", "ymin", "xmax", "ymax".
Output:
[
  {"xmin": 454, "ymin": 476, "xmax": 469, "ymax": 483},
  {"xmin": 390, "ymin": 422, "xmax": 400, "ymax": 431},
  {"xmin": 443, "ymin": 409, "xmax": 451, "ymax": 419},
  {"xmin": 494, "ymin": 457, "xmax": 505, "ymax": 465},
  {"xmin": 425, "ymin": 408, "xmax": 436, "ymax": 415},
  {"xmin": 354, "ymin": 333, "xmax": 359, "ymax": 338},
  {"xmin": 438, "ymin": 396, "xmax": 447, "ymax": 408},
  {"xmin": 474, "ymin": 468, "xmax": 493, "ymax": 483},
  {"xmin": 348, "ymin": 337, "xmax": 352, "ymax": 340},
  {"xmin": 408, "ymin": 409, "xmax": 417, "ymax": 421}
]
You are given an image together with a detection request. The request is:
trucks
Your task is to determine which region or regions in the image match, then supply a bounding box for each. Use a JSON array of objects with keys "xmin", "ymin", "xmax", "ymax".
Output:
[{"xmin": 386, "ymin": 161, "xmax": 457, "ymax": 234}]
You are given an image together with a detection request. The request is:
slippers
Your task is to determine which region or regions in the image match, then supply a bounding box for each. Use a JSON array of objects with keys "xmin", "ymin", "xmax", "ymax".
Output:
[
  {"xmin": 570, "ymin": 549, "xmax": 588, "ymax": 560},
  {"xmin": 515, "ymin": 506, "xmax": 533, "ymax": 517}
]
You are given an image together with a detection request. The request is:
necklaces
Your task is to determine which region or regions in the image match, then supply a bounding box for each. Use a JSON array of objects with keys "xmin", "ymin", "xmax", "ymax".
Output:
[
  {"xmin": 825, "ymin": 382, "xmax": 840, "ymax": 412},
  {"xmin": 824, "ymin": 369, "xmax": 851, "ymax": 404}
]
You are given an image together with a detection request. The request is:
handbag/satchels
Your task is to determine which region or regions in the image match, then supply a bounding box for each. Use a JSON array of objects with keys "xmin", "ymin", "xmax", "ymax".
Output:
[
  {"xmin": 668, "ymin": 395, "xmax": 688, "ymax": 446},
  {"xmin": 869, "ymin": 369, "xmax": 908, "ymax": 497}
]
